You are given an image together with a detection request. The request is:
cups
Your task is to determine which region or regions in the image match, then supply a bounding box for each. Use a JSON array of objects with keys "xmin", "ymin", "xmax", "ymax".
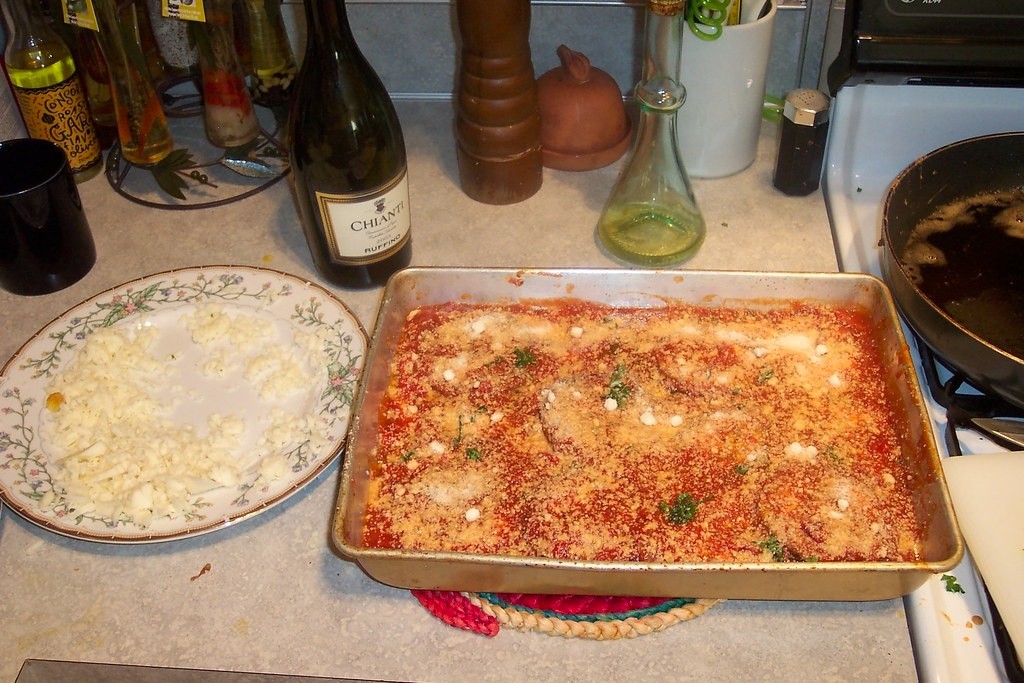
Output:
[
  {"xmin": 648, "ymin": 0, "xmax": 780, "ymax": 181},
  {"xmin": 0, "ymin": 137, "xmax": 98, "ymax": 297}
]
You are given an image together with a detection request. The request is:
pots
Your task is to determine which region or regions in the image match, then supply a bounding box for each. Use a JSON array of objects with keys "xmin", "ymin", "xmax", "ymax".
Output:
[{"xmin": 878, "ymin": 129, "xmax": 1024, "ymax": 407}]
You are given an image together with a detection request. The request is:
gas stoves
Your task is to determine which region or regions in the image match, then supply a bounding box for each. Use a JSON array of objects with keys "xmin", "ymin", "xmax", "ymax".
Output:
[{"xmin": 824, "ymin": 79, "xmax": 1023, "ymax": 683}]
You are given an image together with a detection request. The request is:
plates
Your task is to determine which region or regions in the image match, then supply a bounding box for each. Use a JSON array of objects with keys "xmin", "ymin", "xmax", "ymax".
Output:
[{"xmin": 0, "ymin": 265, "xmax": 372, "ymax": 548}]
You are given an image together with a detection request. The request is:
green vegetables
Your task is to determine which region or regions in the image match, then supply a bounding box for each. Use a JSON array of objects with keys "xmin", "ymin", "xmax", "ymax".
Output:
[{"xmin": 401, "ymin": 348, "xmax": 820, "ymax": 565}]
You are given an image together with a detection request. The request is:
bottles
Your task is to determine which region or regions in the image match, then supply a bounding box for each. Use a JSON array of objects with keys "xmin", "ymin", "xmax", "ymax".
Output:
[
  {"xmin": 771, "ymin": 86, "xmax": 834, "ymax": 198},
  {"xmin": 284, "ymin": 1, "xmax": 413, "ymax": 290},
  {"xmin": 593, "ymin": 1, "xmax": 708, "ymax": 271},
  {"xmin": 1, "ymin": 1, "xmax": 290, "ymax": 177}
]
